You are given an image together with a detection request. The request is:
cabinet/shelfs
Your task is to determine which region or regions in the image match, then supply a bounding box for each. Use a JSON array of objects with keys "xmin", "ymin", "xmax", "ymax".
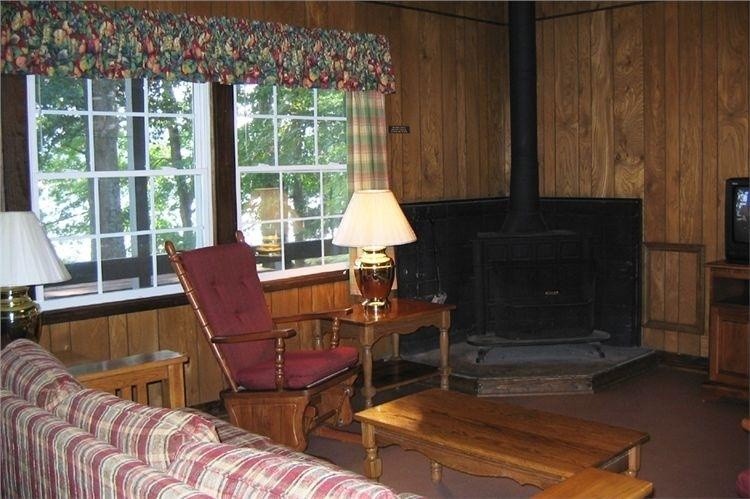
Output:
[{"xmin": 699, "ymin": 259, "xmax": 750, "ymax": 402}]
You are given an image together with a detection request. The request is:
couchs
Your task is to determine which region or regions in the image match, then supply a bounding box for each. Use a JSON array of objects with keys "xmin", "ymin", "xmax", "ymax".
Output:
[{"xmin": 1, "ymin": 333, "xmax": 653, "ymax": 499}]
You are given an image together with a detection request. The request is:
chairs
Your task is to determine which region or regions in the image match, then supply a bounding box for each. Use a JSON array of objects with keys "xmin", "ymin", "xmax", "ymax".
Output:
[{"xmin": 160, "ymin": 229, "xmax": 363, "ymax": 451}]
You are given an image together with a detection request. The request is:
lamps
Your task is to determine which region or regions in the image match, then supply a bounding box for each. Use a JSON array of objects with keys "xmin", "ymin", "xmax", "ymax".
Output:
[
  {"xmin": 0, "ymin": 209, "xmax": 73, "ymax": 345},
  {"xmin": 332, "ymin": 189, "xmax": 419, "ymax": 316}
]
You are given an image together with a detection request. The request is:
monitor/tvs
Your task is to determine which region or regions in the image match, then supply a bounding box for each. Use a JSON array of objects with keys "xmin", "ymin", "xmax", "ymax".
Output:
[{"xmin": 724, "ymin": 177, "xmax": 750, "ymax": 265}]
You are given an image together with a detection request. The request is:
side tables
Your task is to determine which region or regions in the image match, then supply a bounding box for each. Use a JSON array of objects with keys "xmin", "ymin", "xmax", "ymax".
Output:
[{"xmin": 321, "ymin": 299, "xmax": 457, "ymax": 408}]
